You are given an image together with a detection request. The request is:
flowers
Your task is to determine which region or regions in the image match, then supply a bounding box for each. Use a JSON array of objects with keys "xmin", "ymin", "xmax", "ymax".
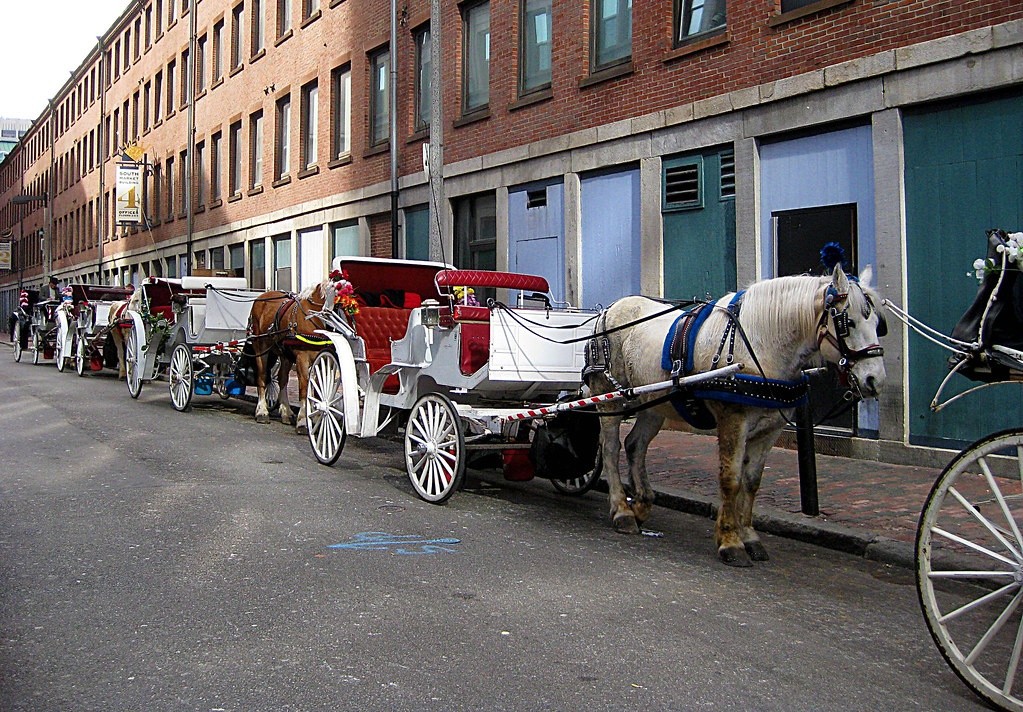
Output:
[
  {"xmin": 329, "ymin": 269, "xmax": 360, "ymax": 315},
  {"xmin": 138, "ymin": 298, "xmax": 171, "ymax": 350},
  {"xmin": 62, "ymin": 287, "xmax": 73, "ymax": 302},
  {"xmin": 453, "ymin": 285, "xmax": 480, "ymax": 307},
  {"xmin": 968, "ymin": 231, "xmax": 1023, "ymax": 286},
  {"xmin": 80, "ymin": 301, "xmax": 89, "ymax": 311}
]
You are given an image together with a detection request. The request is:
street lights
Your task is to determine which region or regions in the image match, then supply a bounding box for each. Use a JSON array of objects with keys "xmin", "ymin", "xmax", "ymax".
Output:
[{"xmin": 13, "ymin": 192, "xmax": 49, "ymax": 282}]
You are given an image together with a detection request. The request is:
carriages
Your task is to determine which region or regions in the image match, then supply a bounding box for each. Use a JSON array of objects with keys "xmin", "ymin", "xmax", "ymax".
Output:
[
  {"xmin": 9, "ymin": 284, "xmax": 152, "ymax": 385},
  {"xmin": 304, "ymin": 256, "xmax": 887, "ymax": 568},
  {"xmin": 122, "ymin": 277, "xmax": 335, "ymax": 435}
]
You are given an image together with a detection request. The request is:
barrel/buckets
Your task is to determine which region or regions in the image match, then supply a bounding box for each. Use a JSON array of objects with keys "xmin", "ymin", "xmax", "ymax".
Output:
[
  {"xmin": 501, "ymin": 442, "xmax": 534, "ymax": 482},
  {"xmin": 420, "ymin": 449, "xmax": 467, "ymax": 488},
  {"xmin": 364, "ymin": 405, "xmax": 398, "ymax": 437},
  {"xmin": 44, "ymin": 347, "xmax": 54, "ymax": 359},
  {"xmin": 193, "ymin": 372, "xmax": 214, "ymax": 395},
  {"xmin": 89, "ymin": 356, "xmax": 104, "ymax": 371},
  {"xmin": 223, "ymin": 374, "xmax": 246, "ymax": 395}
]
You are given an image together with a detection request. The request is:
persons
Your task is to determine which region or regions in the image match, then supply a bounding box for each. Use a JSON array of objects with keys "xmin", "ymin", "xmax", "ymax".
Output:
[
  {"xmin": 39, "ymin": 276, "xmax": 62, "ymax": 302},
  {"xmin": 100, "ymin": 283, "xmax": 134, "ymax": 300}
]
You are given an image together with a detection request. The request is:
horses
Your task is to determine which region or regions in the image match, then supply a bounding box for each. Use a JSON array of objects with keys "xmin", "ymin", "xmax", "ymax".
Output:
[
  {"xmin": 578, "ymin": 263, "xmax": 888, "ymax": 568},
  {"xmin": 107, "ymin": 288, "xmax": 152, "ymax": 384},
  {"xmin": 248, "ymin": 283, "xmax": 329, "ymax": 435}
]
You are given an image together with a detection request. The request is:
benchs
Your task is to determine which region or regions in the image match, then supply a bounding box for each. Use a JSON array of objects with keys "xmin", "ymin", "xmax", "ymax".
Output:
[
  {"xmin": 390, "ymin": 305, "xmax": 452, "ymax": 365},
  {"xmin": 181, "ymin": 276, "xmax": 247, "ymax": 304},
  {"xmin": 435, "ymin": 270, "xmax": 571, "ymax": 324},
  {"xmin": 89, "ymin": 287, "xmax": 133, "ymax": 305},
  {"xmin": 71, "ymin": 306, "xmax": 80, "ymax": 316},
  {"xmin": 150, "ymin": 306, "xmax": 174, "ymax": 321},
  {"xmin": 348, "ymin": 308, "xmax": 412, "ymax": 387}
]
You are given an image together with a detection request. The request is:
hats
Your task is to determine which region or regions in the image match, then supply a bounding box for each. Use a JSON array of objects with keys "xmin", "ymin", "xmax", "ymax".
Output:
[{"xmin": 51, "ymin": 278, "xmax": 59, "ymax": 284}]
